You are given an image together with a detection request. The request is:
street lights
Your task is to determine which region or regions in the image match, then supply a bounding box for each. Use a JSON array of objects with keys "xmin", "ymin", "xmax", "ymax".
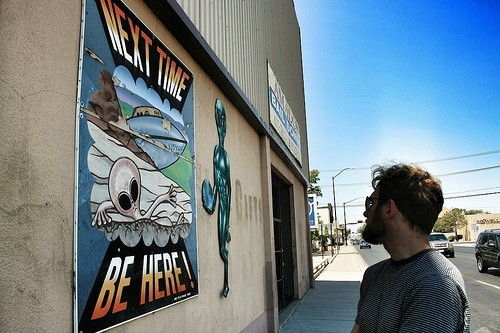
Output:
[
  {"xmin": 332, "ymin": 167, "xmax": 356, "ymax": 250},
  {"xmin": 447, "ymin": 209, "xmax": 458, "ymax": 241},
  {"xmin": 344, "ymin": 195, "xmax": 367, "ymax": 245}
]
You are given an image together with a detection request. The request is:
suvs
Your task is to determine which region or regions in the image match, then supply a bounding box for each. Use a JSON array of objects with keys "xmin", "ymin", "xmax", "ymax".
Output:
[
  {"xmin": 428, "ymin": 232, "xmax": 455, "ymax": 258},
  {"xmin": 360, "ymin": 240, "xmax": 371, "ymax": 250},
  {"xmin": 353, "ymin": 240, "xmax": 359, "ymax": 245},
  {"xmin": 474, "ymin": 229, "xmax": 500, "ymax": 273}
]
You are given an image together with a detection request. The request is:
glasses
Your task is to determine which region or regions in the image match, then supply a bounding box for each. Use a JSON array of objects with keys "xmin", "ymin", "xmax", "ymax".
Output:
[{"xmin": 365, "ymin": 196, "xmax": 387, "ymax": 210}]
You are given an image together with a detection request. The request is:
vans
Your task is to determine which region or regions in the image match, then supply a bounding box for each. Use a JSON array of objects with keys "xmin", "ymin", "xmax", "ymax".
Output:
[{"xmin": 349, "ymin": 238, "xmax": 354, "ymax": 244}]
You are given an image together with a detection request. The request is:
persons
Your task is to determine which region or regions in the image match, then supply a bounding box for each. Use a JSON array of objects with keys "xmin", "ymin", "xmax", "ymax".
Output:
[{"xmin": 351, "ymin": 166, "xmax": 470, "ymax": 333}]
